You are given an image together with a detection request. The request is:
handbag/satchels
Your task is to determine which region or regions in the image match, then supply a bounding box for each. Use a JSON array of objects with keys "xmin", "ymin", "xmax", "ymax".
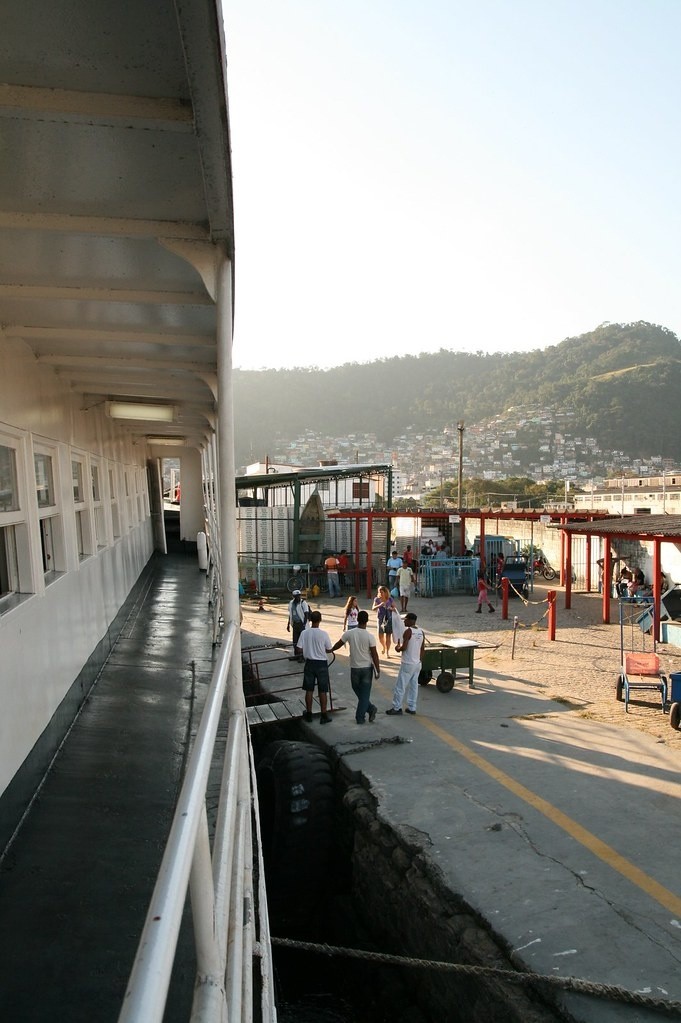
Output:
[
  {"xmin": 392, "ymin": 607, "xmax": 405, "ymax": 644},
  {"xmin": 301, "ymin": 599, "xmax": 312, "ymax": 619},
  {"xmin": 391, "ymin": 586, "xmax": 398, "ymax": 598}
]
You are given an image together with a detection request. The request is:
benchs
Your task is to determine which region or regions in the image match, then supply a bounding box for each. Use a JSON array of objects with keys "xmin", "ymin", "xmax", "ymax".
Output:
[{"xmin": 613, "ymin": 582, "xmax": 652, "ymax": 603}]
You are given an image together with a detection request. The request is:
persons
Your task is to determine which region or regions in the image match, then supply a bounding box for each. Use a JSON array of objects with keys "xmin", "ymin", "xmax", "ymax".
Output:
[
  {"xmin": 420, "ymin": 539, "xmax": 437, "ymax": 556},
  {"xmin": 294, "ymin": 611, "xmax": 335, "ymax": 725},
  {"xmin": 634, "ymin": 571, "xmax": 669, "ymax": 608},
  {"xmin": 287, "ymin": 589, "xmax": 310, "ymax": 664},
  {"xmin": 597, "ymin": 552, "xmax": 630, "ymax": 598},
  {"xmin": 325, "ymin": 610, "xmax": 381, "ymax": 724},
  {"xmin": 616, "ymin": 568, "xmax": 632, "ymax": 598},
  {"xmin": 403, "ymin": 545, "xmax": 413, "ymax": 568},
  {"xmin": 325, "ymin": 550, "xmax": 344, "ymax": 598},
  {"xmin": 343, "ymin": 595, "xmax": 360, "ymax": 656},
  {"xmin": 475, "ymin": 573, "xmax": 495, "ymax": 614},
  {"xmin": 239, "ymin": 582, "xmax": 245, "ymax": 634},
  {"xmin": 395, "ymin": 559, "xmax": 417, "ymax": 613},
  {"xmin": 372, "ymin": 585, "xmax": 398, "ymax": 659},
  {"xmin": 385, "ymin": 550, "xmax": 403, "ymax": 599},
  {"xmin": 385, "ymin": 612, "xmax": 426, "ymax": 715},
  {"xmin": 337, "ymin": 549, "xmax": 349, "ymax": 583},
  {"xmin": 628, "ymin": 567, "xmax": 645, "ymax": 597},
  {"xmin": 433, "ymin": 541, "xmax": 504, "ymax": 579}
]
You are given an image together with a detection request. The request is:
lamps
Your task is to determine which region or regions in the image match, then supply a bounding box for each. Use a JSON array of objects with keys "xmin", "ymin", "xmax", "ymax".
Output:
[
  {"xmin": 146, "ymin": 434, "xmax": 185, "ymax": 446},
  {"xmin": 104, "ymin": 401, "xmax": 179, "ymax": 422}
]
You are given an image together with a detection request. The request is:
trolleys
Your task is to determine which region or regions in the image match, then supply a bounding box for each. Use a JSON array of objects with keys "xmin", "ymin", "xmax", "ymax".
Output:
[
  {"xmin": 615, "ymin": 597, "xmax": 668, "ymax": 714},
  {"xmin": 418, "ymin": 638, "xmax": 504, "ymax": 693}
]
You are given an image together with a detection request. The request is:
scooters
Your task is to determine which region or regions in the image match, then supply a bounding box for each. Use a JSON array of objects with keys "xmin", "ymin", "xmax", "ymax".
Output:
[{"xmin": 498, "ymin": 556, "xmax": 530, "ymax": 598}]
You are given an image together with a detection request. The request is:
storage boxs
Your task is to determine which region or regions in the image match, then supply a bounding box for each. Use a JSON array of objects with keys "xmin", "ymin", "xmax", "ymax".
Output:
[{"xmin": 625, "ymin": 652, "xmax": 660, "ymax": 676}]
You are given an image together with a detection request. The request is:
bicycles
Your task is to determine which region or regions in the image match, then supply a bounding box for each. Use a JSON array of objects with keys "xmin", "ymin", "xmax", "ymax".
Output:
[{"xmin": 286, "ymin": 566, "xmax": 329, "ymax": 593}]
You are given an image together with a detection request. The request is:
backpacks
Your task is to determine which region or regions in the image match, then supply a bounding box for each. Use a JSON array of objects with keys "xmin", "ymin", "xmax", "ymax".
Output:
[{"xmin": 381, "ymin": 609, "xmax": 393, "ymax": 634}]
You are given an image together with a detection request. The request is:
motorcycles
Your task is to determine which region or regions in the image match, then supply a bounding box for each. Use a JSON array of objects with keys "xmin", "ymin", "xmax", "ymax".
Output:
[{"xmin": 523, "ymin": 544, "xmax": 556, "ymax": 580}]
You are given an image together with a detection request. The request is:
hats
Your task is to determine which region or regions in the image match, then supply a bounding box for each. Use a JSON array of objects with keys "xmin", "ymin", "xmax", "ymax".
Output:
[
  {"xmin": 293, "ymin": 590, "xmax": 301, "ymax": 595},
  {"xmin": 402, "ymin": 560, "xmax": 407, "ymax": 563}
]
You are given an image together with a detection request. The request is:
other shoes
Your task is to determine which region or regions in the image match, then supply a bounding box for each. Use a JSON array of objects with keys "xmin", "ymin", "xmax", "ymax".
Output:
[
  {"xmin": 476, "ymin": 610, "xmax": 482, "ymax": 613},
  {"xmin": 387, "ymin": 655, "xmax": 390, "ymax": 658},
  {"xmin": 489, "ymin": 609, "xmax": 495, "ymax": 612},
  {"xmin": 303, "ymin": 710, "xmax": 312, "ymax": 722},
  {"xmin": 358, "ymin": 719, "xmax": 365, "ymax": 724},
  {"xmin": 369, "ymin": 706, "xmax": 377, "ymax": 722},
  {"xmin": 405, "ymin": 709, "xmax": 416, "ymax": 714},
  {"xmin": 382, "ymin": 649, "xmax": 385, "ymax": 654},
  {"xmin": 320, "ymin": 713, "xmax": 332, "ymax": 724},
  {"xmin": 386, "ymin": 708, "xmax": 403, "ymax": 715}
]
